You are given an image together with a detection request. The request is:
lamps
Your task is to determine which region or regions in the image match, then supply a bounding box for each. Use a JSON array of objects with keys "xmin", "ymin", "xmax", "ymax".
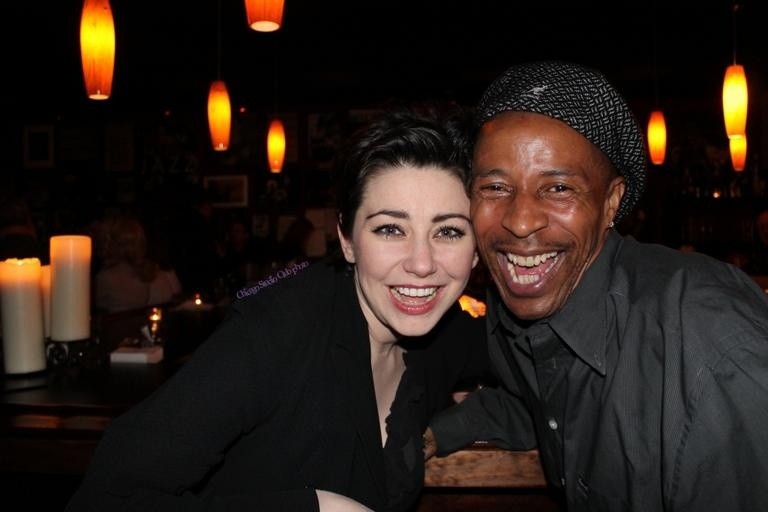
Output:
[
  {"xmin": 80, "ymin": 0, "xmax": 286, "ymax": 174},
  {"xmin": 647, "ymin": 0, "xmax": 750, "ymax": 173}
]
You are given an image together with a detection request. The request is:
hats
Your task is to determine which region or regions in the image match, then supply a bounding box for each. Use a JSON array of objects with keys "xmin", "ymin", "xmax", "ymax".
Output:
[{"xmin": 472, "ymin": 61, "xmax": 647, "ymax": 225}]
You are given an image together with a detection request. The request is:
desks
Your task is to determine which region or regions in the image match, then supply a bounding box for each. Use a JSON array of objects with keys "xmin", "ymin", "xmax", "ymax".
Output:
[{"xmin": 0, "ymin": 361, "xmax": 175, "ymax": 474}]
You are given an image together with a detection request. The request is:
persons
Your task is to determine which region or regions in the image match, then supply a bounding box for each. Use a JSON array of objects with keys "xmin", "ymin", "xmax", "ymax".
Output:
[
  {"xmin": 417, "ymin": 59, "xmax": 768, "ymax": 511},
  {"xmin": 60, "ymin": 105, "xmax": 489, "ymax": 511},
  {"xmin": 726, "ymin": 211, "xmax": 768, "ymax": 277},
  {"xmin": 1, "ymin": 186, "xmax": 315, "ymax": 315},
  {"xmin": 677, "ymin": 241, "xmax": 695, "ymax": 253}
]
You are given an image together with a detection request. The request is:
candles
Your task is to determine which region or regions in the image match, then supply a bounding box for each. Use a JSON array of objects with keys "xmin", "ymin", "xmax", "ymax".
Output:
[{"xmin": 0, "ymin": 235, "xmax": 91, "ymax": 374}]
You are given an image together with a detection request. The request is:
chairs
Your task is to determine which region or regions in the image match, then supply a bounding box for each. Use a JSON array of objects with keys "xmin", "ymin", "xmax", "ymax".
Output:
[{"xmin": 91, "ymin": 301, "xmax": 178, "ymax": 339}]
